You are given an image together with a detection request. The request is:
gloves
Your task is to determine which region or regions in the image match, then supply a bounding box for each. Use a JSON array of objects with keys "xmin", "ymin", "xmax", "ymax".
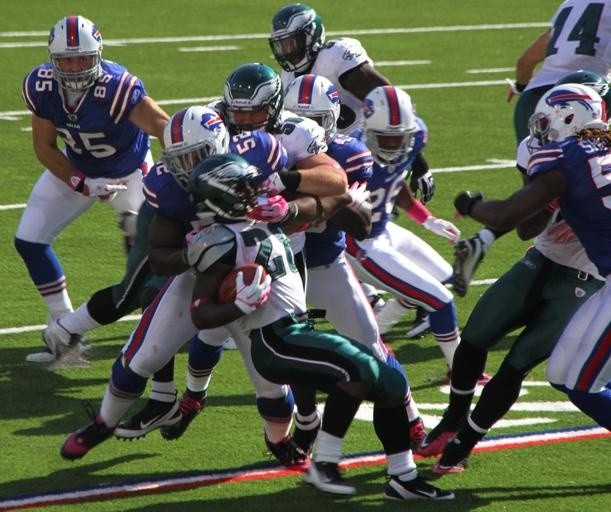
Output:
[
  {"xmin": 409, "ymin": 201, "xmax": 463, "ymax": 251},
  {"xmin": 245, "ymin": 191, "xmax": 297, "ymax": 223},
  {"xmin": 343, "ymin": 179, "xmax": 370, "ymax": 218},
  {"xmin": 68, "ymin": 169, "xmax": 131, "ymax": 202},
  {"xmin": 230, "ymin": 263, "xmax": 273, "ymax": 317}
]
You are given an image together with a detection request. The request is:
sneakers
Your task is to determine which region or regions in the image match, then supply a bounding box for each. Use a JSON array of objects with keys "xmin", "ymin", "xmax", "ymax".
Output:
[
  {"xmin": 61, "ymin": 414, "xmax": 114, "ymax": 464},
  {"xmin": 448, "ymin": 233, "xmax": 493, "ymax": 301},
  {"xmin": 31, "ymin": 312, "xmax": 95, "ymax": 369},
  {"xmin": 160, "ymin": 383, "xmax": 206, "ymax": 449},
  {"xmin": 409, "ymin": 416, "xmax": 474, "ymax": 473},
  {"xmin": 360, "ymin": 284, "xmax": 496, "ymax": 391},
  {"xmin": 380, "ymin": 467, "xmax": 451, "ymax": 502},
  {"xmin": 291, "ymin": 407, "xmax": 322, "ymax": 454},
  {"xmin": 309, "ymin": 444, "xmax": 355, "ymax": 498},
  {"xmin": 110, "ymin": 392, "xmax": 184, "ymax": 442},
  {"xmin": 262, "ymin": 429, "xmax": 308, "ymax": 484}
]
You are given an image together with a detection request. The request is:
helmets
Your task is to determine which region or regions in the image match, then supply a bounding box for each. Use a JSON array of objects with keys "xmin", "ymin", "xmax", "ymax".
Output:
[
  {"xmin": 287, "ymin": 75, "xmax": 343, "ymax": 136},
  {"xmin": 157, "ymin": 104, "xmax": 231, "ymax": 182},
  {"xmin": 40, "ymin": 16, "xmax": 106, "ymax": 91},
  {"xmin": 221, "ymin": 62, "xmax": 278, "ymax": 131},
  {"xmin": 187, "ymin": 150, "xmax": 256, "ymax": 220},
  {"xmin": 516, "ymin": 70, "xmax": 611, "ymax": 174},
  {"xmin": 266, "ymin": 3, "xmax": 318, "ymax": 74},
  {"xmin": 361, "ymin": 85, "xmax": 415, "ymax": 165}
]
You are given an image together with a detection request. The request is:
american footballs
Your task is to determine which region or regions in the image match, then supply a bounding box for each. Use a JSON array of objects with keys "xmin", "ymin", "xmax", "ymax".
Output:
[{"xmin": 219, "ymin": 263, "xmax": 267, "ymax": 305}]
